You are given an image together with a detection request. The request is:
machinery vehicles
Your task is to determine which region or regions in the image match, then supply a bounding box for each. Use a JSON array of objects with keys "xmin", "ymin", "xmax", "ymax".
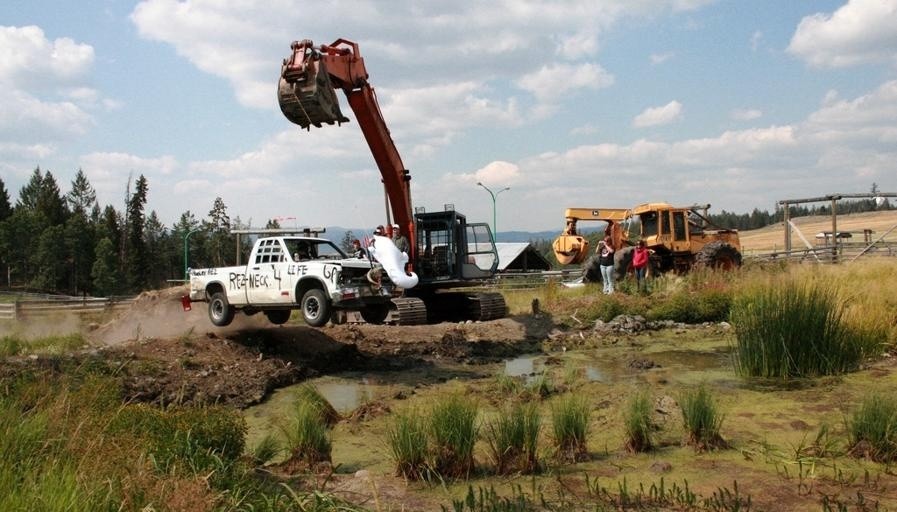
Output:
[
  {"xmin": 277, "ymin": 36, "xmax": 509, "ymax": 323},
  {"xmin": 552, "ymin": 202, "xmax": 741, "ymax": 284}
]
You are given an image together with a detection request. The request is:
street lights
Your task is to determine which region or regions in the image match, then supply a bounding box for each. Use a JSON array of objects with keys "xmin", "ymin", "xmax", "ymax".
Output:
[
  {"xmin": 175, "ymin": 221, "xmax": 201, "ymax": 280},
  {"xmin": 475, "ymin": 182, "xmax": 509, "ymax": 242}
]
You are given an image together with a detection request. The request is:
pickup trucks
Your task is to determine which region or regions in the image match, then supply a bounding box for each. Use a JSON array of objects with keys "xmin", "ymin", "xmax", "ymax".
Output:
[{"xmin": 190, "ymin": 234, "xmax": 393, "ymax": 326}]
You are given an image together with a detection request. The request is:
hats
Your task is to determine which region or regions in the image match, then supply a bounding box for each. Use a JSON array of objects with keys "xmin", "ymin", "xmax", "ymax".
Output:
[{"xmin": 391, "ymin": 224, "xmax": 400, "ymax": 229}]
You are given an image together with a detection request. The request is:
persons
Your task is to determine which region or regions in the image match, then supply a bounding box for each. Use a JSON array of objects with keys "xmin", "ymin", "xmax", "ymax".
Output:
[
  {"xmin": 292, "ymin": 241, "xmax": 311, "ymax": 259},
  {"xmin": 595, "ymin": 235, "xmax": 615, "ymax": 295},
  {"xmin": 632, "ymin": 240, "xmax": 649, "ymax": 293},
  {"xmin": 351, "ymin": 224, "xmax": 410, "ymax": 262}
]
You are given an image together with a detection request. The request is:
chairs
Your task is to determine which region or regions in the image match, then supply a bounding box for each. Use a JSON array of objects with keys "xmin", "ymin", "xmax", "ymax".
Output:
[{"xmin": 432, "ymin": 245, "xmax": 450, "ymax": 274}]
